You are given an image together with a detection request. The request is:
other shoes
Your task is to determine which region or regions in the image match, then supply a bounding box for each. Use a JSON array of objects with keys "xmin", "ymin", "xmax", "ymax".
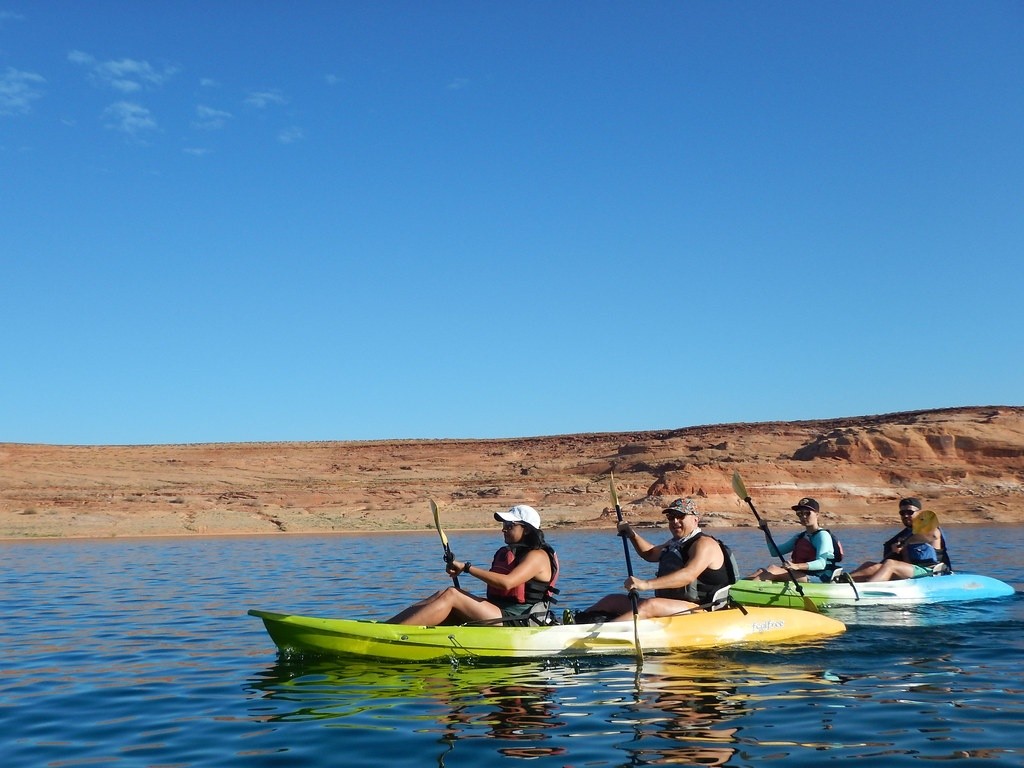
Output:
[{"xmin": 563, "ymin": 609, "xmax": 576, "ymax": 625}]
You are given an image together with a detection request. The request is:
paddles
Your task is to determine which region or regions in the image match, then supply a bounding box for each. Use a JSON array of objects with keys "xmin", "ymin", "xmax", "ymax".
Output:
[
  {"xmin": 731, "ymin": 469, "xmax": 823, "ymax": 616},
  {"xmin": 428, "ymin": 498, "xmax": 462, "ymax": 590},
  {"xmin": 607, "ymin": 469, "xmax": 645, "ymax": 670},
  {"xmin": 876, "ymin": 509, "xmax": 940, "ymax": 564}
]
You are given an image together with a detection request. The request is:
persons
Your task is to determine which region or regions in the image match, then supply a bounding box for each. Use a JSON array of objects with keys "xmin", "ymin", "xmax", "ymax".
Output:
[
  {"xmin": 564, "ymin": 499, "xmax": 734, "ymax": 622},
  {"xmin": 742, "ymin": 499, "xmax": 836, "ymax": 587},
  {"xmin": 847, "ymin": 497, "xmax": 944, "ymax": 584},
  {"xmin": 384, "ymin": 504, "xmax": 556, "ymax": 628}
]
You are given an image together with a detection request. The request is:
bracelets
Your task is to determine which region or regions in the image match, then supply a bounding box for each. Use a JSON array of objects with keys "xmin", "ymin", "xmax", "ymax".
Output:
[{"xmin": 463, "ymin": 562, "xmax": 472, "ymax": 573}]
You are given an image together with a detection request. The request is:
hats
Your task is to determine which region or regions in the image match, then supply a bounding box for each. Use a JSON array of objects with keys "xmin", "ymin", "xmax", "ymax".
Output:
[
  {"xmin": 792, "ymin": 498, "xmax": 819, "ymax": 513},
  {"xmin": 900, "ymin": 498, "xmax": 921, "ymax": 510},
  {"xmin": 662, "ymin": 498, "xmax": 699, "ymax": 515},
  {"xmin": 494, "ymin": 504, "xmax": 541, "ymax": 529}
]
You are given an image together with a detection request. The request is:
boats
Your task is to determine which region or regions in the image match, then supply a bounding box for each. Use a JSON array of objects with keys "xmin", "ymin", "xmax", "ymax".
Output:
[
  {"xmin": 245, "ymin": 605, "xmax": 848, "ymax": 664},
  {"xmin": 726, "ymin": 572, "xmax": 1019, "ymax": 606}
]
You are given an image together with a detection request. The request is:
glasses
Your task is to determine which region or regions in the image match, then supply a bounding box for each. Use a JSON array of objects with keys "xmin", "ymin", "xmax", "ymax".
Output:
[
  {"xmin": 666, "ymin": 511, "xmax": 694, "ymax": 521},
  {"xmin": 796, "ymin": 510, "xmax": 813, "ymax": 517},
  {"xmin": 899, "ymin": 510, "xmax": 918, "ymax": 516},
  {"xmin": 501, "ymin": 520, "xmax": 526, "ymax": 530}
]
